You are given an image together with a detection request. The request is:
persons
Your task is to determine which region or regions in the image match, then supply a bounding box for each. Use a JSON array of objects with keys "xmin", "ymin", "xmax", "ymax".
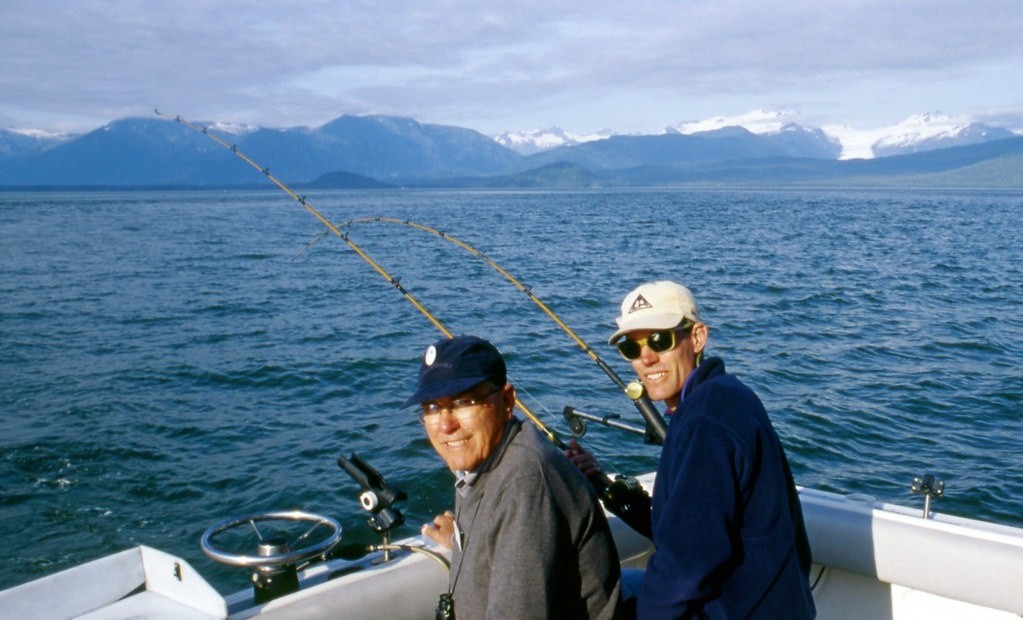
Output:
[
  {"xmin": 608, "ymin": 277, "xmax": 819, "ymax": 620},
  {"xmin": 404, "ymin": 336, "xmax": 634, "ymax": 620}
]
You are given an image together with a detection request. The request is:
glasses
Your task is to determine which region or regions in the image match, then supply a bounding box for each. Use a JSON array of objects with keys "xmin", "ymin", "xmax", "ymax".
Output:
[
  {"xmin": 615, "ymin": 322, "xmax": 693, "ymax": 361},
  {"xmin": 414, "ymin": 388, "xmax": 503, "ymax": 424}
]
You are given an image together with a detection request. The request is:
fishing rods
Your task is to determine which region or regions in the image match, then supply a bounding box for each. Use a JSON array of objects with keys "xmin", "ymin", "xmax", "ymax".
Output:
[
  {"xmin": 296, "ymin": 216, "xmax": 670, "ymax": 449},
  {"xmin": 155, "ymin": 108, "xmax": 655, "ymax": 546}
]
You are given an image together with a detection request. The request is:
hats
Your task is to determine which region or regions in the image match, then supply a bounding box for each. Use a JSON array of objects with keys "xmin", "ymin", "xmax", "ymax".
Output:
[
  {"xmin": 399, "ymin": 335, "xmax": 507, "ymax": 411},
  {"xmin": 608, "ymin": 280, "xmax": 700, "ymax": 346}
]
